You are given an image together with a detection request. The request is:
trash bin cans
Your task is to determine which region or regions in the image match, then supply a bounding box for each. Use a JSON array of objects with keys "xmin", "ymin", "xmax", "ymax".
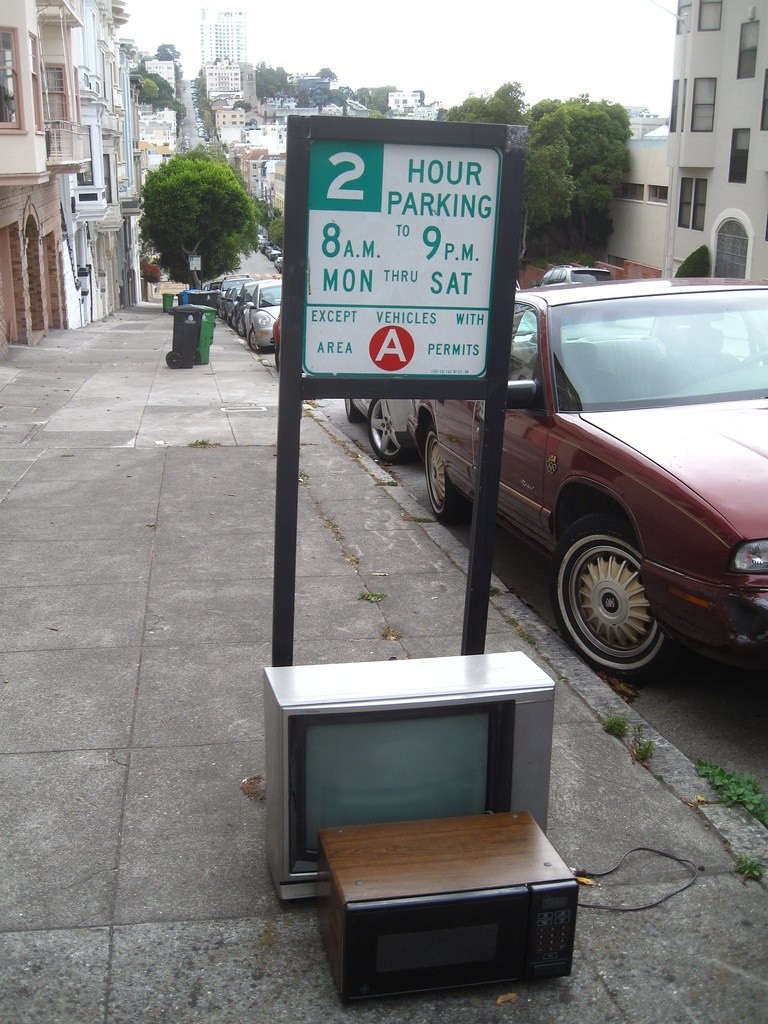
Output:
[
  {"xmin": 165, "ymin": 306, "xmax": 204, "ymax": 369},
  {"xmin": 192, "ymin": 305, "xmax": 217, "ymax": 365},
  {"xmin": 161, "ymin": 292, "xmax": 175, "ymax": 312},
  {"xmin": 175, "ymin": 288, "xmax": 221, "ymax": 316}
]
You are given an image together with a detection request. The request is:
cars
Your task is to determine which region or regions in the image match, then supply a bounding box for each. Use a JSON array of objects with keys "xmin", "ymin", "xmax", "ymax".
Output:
[
  {"xmin": 340, "ymin": 398, "xmax": 417, "ymax": 463},
  {"xmin": 203, "ymin": 235, "xmax": 280, "ymax": 369},
  {"xmin": 408, "ymin": 274, "xmax": 768, "ymax": 683}
]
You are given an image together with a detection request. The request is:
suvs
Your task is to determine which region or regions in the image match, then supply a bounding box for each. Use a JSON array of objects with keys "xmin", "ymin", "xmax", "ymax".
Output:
[{"xmin": 531, "ymin": 264, "xmax": 612, "ymax": 292}]
